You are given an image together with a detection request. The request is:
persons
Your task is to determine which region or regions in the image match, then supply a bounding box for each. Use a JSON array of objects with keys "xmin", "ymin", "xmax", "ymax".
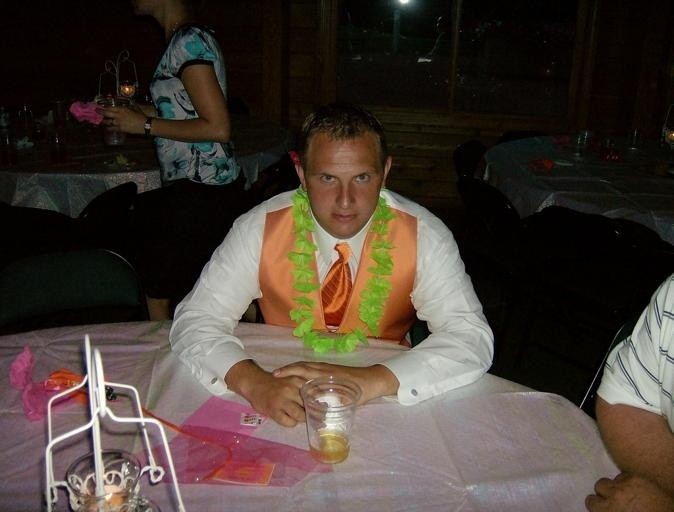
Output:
[
  {"xmin": 96, "ymin": 0, "xmax": 244, "ymax": 321},
  {"xmin": 585, "ymin": 274, "xmax": 673, "ymax": 512},
  {"xmin": 169, "ymin": 102, "xmax": 494, "ymax": 427}
]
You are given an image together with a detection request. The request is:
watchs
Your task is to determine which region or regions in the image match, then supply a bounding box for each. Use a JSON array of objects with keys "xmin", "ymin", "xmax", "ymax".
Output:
[{"xmin": 145, "ymin": 117, "xmax": 153, "ymax": 136}]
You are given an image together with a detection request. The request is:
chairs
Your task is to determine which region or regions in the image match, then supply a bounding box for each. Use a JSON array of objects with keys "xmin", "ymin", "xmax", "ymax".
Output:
[
  {"xmin": 71, "ymin": 180, "xmax": 142, "ymax": 236},
  {"xmin": 452, "ymin": 124, "xmax": 515, "ymax": 215},
  {"xmin": 0, "ymin": 246, "xmax": 144, "ymax": 336},
  {"xmin": 498, "ymin": 128, "xmax": 542, "ymax": 143},
  {"xmin": 579, "ymin": 320, "xmax": 640, "ymax": 420}
]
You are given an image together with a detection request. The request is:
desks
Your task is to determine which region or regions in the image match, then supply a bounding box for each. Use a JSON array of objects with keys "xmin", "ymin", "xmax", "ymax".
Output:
[
  {"xmin": 485, "ymin": 134, "xmax": 674, "ymax": 249},
  {"xmin": 1, "ymin": 320, "xmax": 622, "ymax": 512},
  {"xmin": 1, "ymin": 118, "xmax": 288, "ymax": 215}
]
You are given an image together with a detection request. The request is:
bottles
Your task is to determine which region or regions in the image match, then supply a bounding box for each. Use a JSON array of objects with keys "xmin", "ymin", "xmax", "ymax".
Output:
[
  {"xmin": 553, "ymin": 124, "xmax": 639, "ymax": 167},
  {"xmin": 0, "ymin": 105, "xmax": 11, "ymax": 147},
  {"xmin": 54, "ymin": 100, "xmax": 64, "ymax": 146},
  {"xmin": 16, "ymin": 105, "xmax": 34, "ymax": 137}
]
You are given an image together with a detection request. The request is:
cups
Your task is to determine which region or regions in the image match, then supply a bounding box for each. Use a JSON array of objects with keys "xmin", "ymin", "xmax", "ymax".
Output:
[
  {"xmin": 64, "ymin": 447, "xmax": 161, "ymax": 511},
  {"xmin": 96, "ymin": 97, "xmax": 130, "ymax": 144},
  {"xmin": 298, "ymin": 375, "xmax": 362, "ymax": 464}
]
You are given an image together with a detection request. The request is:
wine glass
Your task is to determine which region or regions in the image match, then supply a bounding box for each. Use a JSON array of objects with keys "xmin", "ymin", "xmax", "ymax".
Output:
[{"xmin": 119, "ymin": 82, "xmax": 135, "ymax": 105}]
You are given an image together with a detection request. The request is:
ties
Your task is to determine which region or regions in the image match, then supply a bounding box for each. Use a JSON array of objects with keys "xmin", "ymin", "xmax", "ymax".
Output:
[{"xmin": 321, "ymin": 243, "xmax": 351, "ymax": 331}]
